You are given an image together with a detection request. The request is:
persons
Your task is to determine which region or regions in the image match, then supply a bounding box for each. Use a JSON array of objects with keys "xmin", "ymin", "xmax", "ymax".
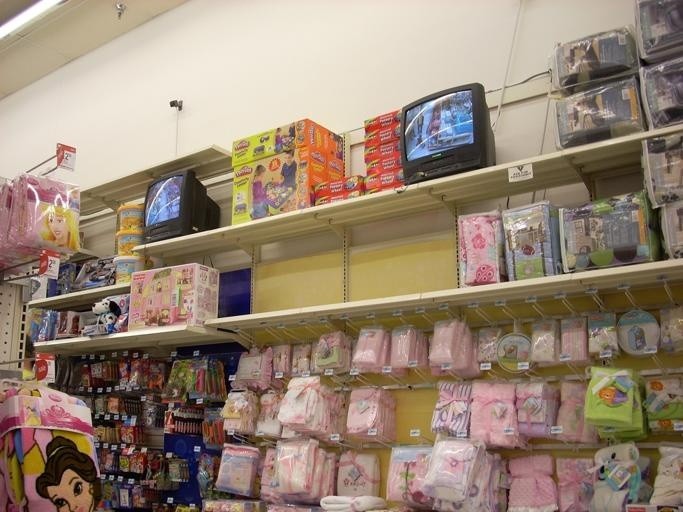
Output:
[
  {"xmin": 252, "ymin": 164, "xmax": 274, "ymax": 218},
  {"xmin": 288, "ymin": 123, "xmax": 296, "ymax": 141},
  {"xmin": 40, "ymin": 206, "xmax": 81, "ymax": 251},
  {"xmin": 275, "ymin": 127, "xmax": 284, "ymax": 152},
  {"xmin": 278, "ymin": 149, "xmax": 296, "ymax": 190}
]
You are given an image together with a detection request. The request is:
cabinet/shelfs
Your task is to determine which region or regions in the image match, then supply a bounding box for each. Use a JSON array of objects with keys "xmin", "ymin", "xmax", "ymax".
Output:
[{"xmin": 0, "ymin": 76, "xmax": 683, "ymax": 512}]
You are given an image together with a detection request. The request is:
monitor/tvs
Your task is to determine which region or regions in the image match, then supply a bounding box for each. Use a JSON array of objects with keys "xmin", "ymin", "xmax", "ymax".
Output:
[
  {"xmin": 400, "ymin": 81, "xmax": 496, "ymax": 186},
  {"xmin": 141, "ymin": 169, "xmax": 221, "ymax": 247}
]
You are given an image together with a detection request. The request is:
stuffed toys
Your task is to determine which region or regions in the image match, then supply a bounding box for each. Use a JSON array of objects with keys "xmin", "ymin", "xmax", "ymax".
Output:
[{"xmin": 89, "ymin": 298, "xmax": 122, "ymax": 334}]
